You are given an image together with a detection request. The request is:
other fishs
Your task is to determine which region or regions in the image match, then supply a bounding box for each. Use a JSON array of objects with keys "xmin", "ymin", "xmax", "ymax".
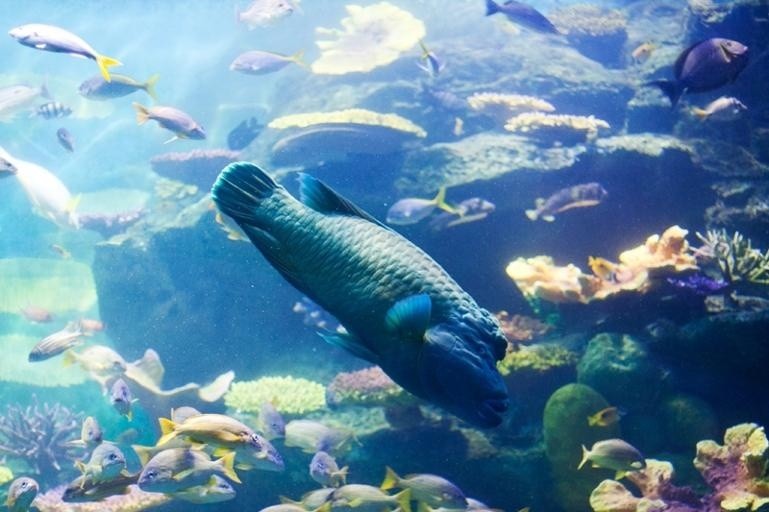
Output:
[
  {"xmin": 0, "ymin": 0, "xmax": 303, "ymax": 232},
  {"xmin": 0, "ymin": 304, "xmax": 493, "ymax": 511}
]
